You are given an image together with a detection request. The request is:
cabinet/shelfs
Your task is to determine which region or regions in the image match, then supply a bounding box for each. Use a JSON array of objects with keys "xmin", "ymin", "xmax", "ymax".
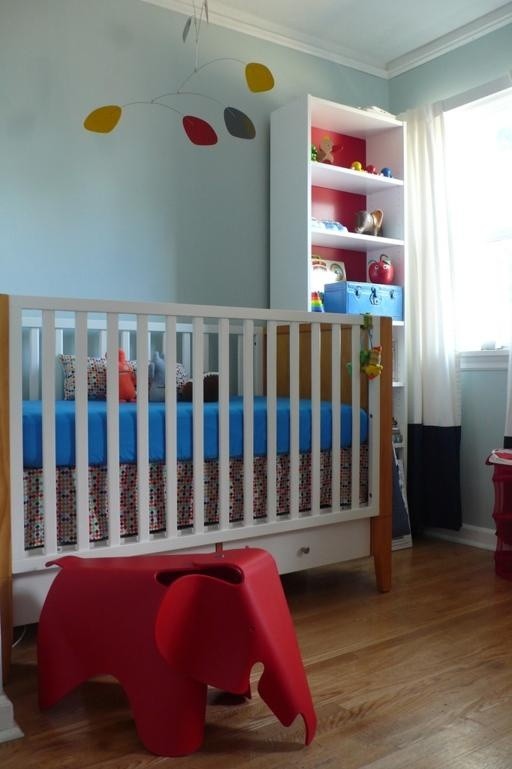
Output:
[{"xmin": 269, "ymin": 91, "xmax": 419, "ymax": 553}]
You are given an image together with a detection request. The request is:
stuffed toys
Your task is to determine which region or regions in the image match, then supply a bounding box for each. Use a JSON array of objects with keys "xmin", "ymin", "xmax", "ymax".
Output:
[
  {"xmin": 362, "ymin": 347, "xmax": 384, "ymax": 381},
  {"xmin": 105, "ymin": 346, "xmax": 137, "ymax": 402},
  {"xmin": 347, "ymin": 351, "xmax": 366, "ymax": 377},
  {"xmin": 152, "ymin": 352, "xmax": 167, "ymax": 403},
  {"xmin": 181, "ymin": 371, "xmax": 219, "ymax": 402}
]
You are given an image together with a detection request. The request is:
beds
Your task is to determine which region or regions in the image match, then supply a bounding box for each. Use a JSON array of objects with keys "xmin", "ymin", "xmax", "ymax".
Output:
[{"xmin": 0, "ymin": 290, "xmax": 393, "ymax": 694}]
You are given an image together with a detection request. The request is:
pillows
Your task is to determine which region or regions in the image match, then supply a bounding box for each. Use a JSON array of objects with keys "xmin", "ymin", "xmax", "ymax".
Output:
[{"xmin": 56, "ymin": 351, "xmax": 189, "ymax": 404}]
